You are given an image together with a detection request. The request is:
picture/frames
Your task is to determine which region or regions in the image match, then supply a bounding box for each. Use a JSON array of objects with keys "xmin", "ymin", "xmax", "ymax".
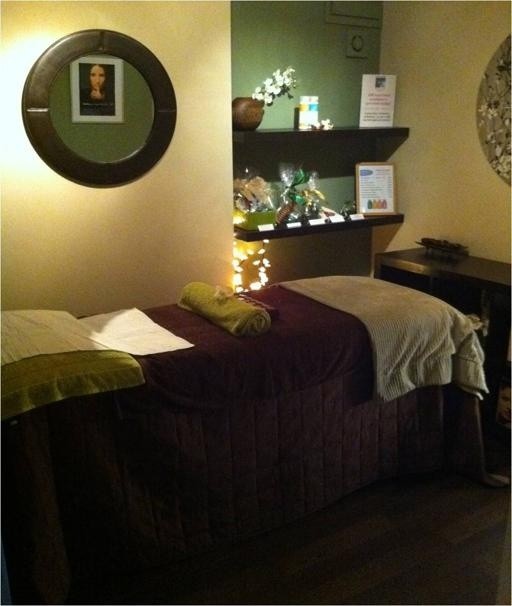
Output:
[
  {"xmin": 356, "ymin": 161, "xmax": 398, "ymax": 216},
  {"xmin": 69, "ymin": 58, "xmax": 126, "ymax": 125}
]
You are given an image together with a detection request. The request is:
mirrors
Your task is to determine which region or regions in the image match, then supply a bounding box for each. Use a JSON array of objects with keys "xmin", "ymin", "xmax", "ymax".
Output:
[{"xmin": 21, "ymin": 28, "xmax": 176, "ymax": 189}]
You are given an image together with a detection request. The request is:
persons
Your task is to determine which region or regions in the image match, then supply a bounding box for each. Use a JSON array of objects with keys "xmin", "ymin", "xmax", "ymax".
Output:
[{"xmin": 79, "ymin": 62, "xmax": 116, "ymax": 115}]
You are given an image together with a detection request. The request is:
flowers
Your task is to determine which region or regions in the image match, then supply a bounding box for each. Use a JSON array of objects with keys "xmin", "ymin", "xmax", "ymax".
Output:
[
  {"xmin": 232, "ymin": 231, "xmax": 271, "ymax": 294},
  {"xmin": 252, "ymin": 65, "xmax": 297, "ymax": 105}
]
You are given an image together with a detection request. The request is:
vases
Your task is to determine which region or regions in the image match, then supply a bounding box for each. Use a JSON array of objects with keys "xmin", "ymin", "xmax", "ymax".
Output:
[{"xmin": 232, "ymin": 97, "xmax": 264, "ymax": 129}]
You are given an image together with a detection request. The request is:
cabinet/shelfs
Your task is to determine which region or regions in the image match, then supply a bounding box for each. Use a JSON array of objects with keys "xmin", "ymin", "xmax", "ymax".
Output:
[{"xmin": 233, "ymin": 125, "xmax": 409, "ymax": 241}]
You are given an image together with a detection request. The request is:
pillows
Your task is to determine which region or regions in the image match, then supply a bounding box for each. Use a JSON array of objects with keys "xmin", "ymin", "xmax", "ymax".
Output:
[{"xmin": 1, "ymin": 307, "xmax": 144, "ymax": 421}]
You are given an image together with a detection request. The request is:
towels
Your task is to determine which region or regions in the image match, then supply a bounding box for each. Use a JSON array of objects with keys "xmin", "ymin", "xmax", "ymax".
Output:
[
  {"xmin": 272, "ymin": 277, "xmax": 490, "ymax": 406},
  {"xmin": 175, "ymin": 280, "xmax": 271, "ymax": 339},
  {"xmin": 1, "ymin": 306, "xmax": 196, "ymax": 369}
]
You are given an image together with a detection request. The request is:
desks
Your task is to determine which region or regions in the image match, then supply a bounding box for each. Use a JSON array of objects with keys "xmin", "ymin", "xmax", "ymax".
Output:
[{"xmin": 374, "ymin": 247, "xmax": 510, "ymax": 318}]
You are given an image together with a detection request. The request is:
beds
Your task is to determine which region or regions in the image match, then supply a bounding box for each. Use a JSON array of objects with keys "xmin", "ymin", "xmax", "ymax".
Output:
[{"xmin": 1, "ymin": 275, "xmax": 490, "ymax": 606}]
